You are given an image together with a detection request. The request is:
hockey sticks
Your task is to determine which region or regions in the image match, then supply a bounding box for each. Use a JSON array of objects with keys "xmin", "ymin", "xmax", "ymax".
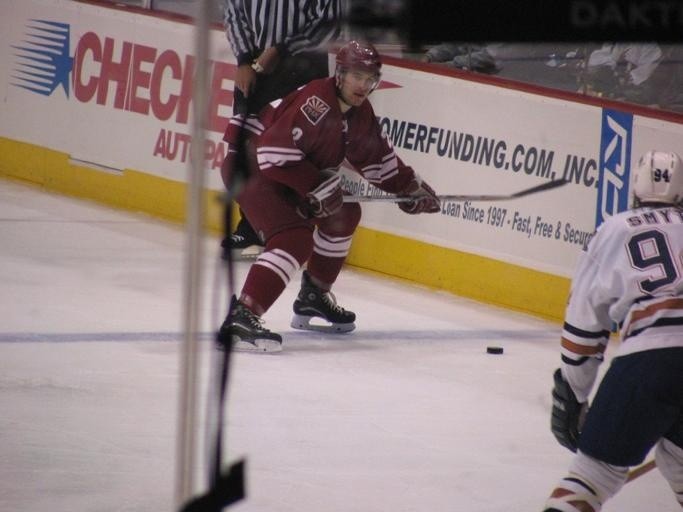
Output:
[{"xmin": 310, "ymin": 178, "xmax": 569, "ymax": 206}]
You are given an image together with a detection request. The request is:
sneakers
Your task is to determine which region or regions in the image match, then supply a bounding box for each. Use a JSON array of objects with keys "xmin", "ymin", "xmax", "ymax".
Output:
[
  {"xmin": 293, "ymin": 270, "xmax": 356, "ymax": 323},
  {"xmin": 221, "ymin": 229, "xmax": 264, "ymax": 249},
  {"xmin": 218, "ymin": 294, "xmax": 282, "ymax": 345}
]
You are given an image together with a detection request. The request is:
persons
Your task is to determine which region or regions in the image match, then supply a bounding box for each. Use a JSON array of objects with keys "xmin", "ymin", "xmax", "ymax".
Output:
[
  {"xmin": 215, "ymin": 38, "xmax": 440, "ymax": 351},
  {"xmin": 221, "ymin": 0, "xmax": 356, "ymax": 261},
  {"xmin": 540, "ymin": 148, "xmax": 683, "ymax": 512}
]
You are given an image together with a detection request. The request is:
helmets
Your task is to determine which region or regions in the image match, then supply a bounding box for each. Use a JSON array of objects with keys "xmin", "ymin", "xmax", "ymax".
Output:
[
  {"xmin": 334, "ymin": 39, "xmax": 383, "ymax": 74},
  {"xmin": 631, "ymin": 149, "xmax": 682, "ymax": 205}
]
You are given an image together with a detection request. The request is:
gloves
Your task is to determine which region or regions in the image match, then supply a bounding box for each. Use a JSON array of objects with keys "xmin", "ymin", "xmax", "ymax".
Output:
[
  {"xmin": 549, "ymin": 367, "xmax": 589, "ymax": 453},
  {"xmin": 395, "ymin": 177, "xmax": 442, "ymax": 215},
  {"xmin": 306, "ymin": 168, "xmax": 344, "ymax": 220}
]
